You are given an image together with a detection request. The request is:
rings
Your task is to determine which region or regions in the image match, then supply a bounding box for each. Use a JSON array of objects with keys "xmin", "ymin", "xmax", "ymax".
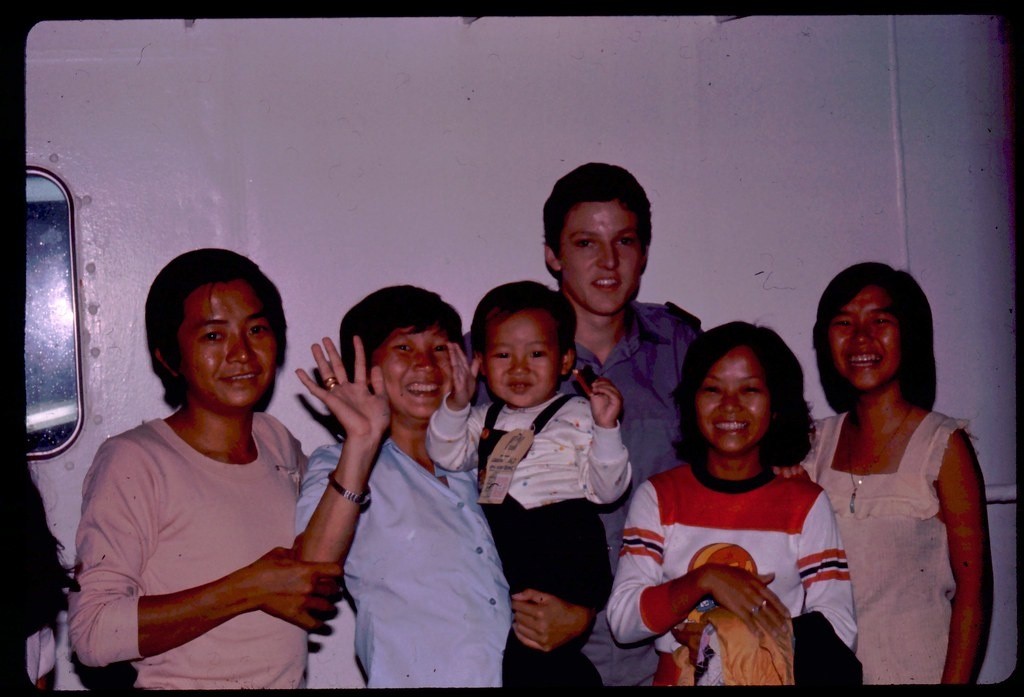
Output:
[
  {"xmin": 327, "ymin": 382, "xmax": 338, "ymax": 390},
  {"xmin": 752, "ymin": 606, "xmax": 761, "ymax": 614},
  {"xmin": 324, "ymin": 377, "xmax": 336, "ymax": 384},
  {"xmin": 761, "ymin": 600, "xmax": 769, "ymax": 608}
]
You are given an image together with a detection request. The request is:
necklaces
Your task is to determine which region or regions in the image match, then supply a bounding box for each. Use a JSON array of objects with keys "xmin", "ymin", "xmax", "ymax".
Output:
[{"xmin": 849, "ymin": 406, "xmax": 913, "ymax": 513}]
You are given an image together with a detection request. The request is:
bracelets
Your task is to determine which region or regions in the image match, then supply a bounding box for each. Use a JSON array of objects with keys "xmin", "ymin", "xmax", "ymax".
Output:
[{"xmin": 328, "ymin": 474, "xmax": 369, "ymax": 505}]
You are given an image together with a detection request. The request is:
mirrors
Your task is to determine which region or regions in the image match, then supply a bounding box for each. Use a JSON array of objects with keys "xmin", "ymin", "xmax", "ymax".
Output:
[{"xmin": 23, "ymin": 167, "xmax": 84, "ymax": 458}]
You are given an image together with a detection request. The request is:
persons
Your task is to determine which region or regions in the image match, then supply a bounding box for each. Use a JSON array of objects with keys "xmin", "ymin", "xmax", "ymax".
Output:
[
  {"xmin": 607, "ymin": 321, "xmax": 856, "ymax": 683},
  {"xmin": 292, "ymin": 285, "xmax": 596, "ymax": 687},
  {"xmin": 465, "ymin": 163, "xmax": 703, "ymax": 686},
  {"xmin": 26, "ymin": 469, "xmax": 81, "ymax": 688},
  {"xmin": 69, "ymin": 249, "xmax": 344, "ymax": 688},
  {"xmin": 773, "ymin": 263, "xmax": 993, "ymax": 683},
  {"xmin": 425, "ymin": 281, "xmax": 634, "ymax": 687}
]
